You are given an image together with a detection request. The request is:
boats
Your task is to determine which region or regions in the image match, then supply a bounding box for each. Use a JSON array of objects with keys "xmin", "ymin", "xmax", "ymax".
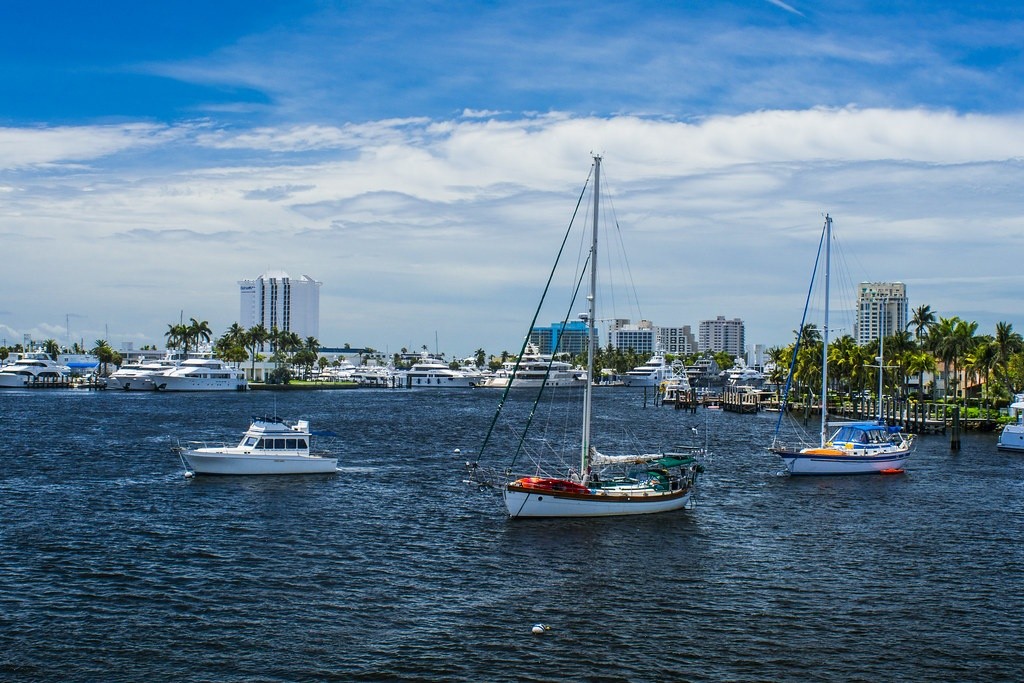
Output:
[
  {"xmin": 148, "ymin": 359, "xmax": 250, "ymax": 391},
  {"xmin": 616, "ymin": 350, "xmax": 688, "ymax": 388},
  {"xmin": 107, "ymin": 354, "xmax": 185, "ymax": 391},
  {"xmin": 169, "ymin": 413, "xmax": 340, "ymax": 475},
  {"xmin": 395, "ymin": 358, "xmax": 486, "ymax": 388},
  {"xmin": 995, "ymin": 400, "xmax": 1024, "ymax": 454},
  {"xmin": 0, "ymin": 349, "xmax": 71, "ymax": 389},
  {"xmin": 485, "ymin": 337, "xmax": 589, "ymax": 388}
]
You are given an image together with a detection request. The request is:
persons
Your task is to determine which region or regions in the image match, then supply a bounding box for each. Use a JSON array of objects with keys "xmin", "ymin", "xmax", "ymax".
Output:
[
  {"xmin": 587, "ymin": 473, "xmax": 602, "ymax": 489},
  {"xmin": 860, "ymin": 430, "xmax": 869, "ymax": 443},
  {"xmin": 675, "ymin": 469, "xmax": 687, "ymax": 489}
]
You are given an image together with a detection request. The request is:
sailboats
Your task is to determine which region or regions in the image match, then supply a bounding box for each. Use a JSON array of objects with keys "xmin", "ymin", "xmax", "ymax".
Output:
[
  {"xmin": 765, "ymin": 209, "xmax": 918, "ymax": 476},
  {"xmin": 462, "ymin": 154, "xmax": 709, "ymax": 518}
]
